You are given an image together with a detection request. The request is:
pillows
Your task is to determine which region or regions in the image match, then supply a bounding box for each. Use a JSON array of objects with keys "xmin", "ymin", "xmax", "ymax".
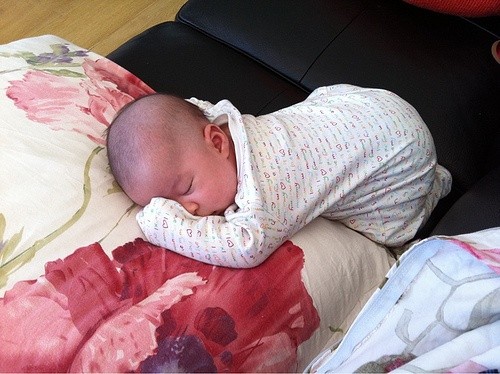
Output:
[{"xmin": 0, "ymin": 34, "xmax": 393, "ymax": 374}]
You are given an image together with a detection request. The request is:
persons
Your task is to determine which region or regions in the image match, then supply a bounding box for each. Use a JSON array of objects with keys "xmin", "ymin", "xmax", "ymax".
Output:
[{"xmin": 106, "ymin": 84, "xmax": 453, "ymax": 268}]
